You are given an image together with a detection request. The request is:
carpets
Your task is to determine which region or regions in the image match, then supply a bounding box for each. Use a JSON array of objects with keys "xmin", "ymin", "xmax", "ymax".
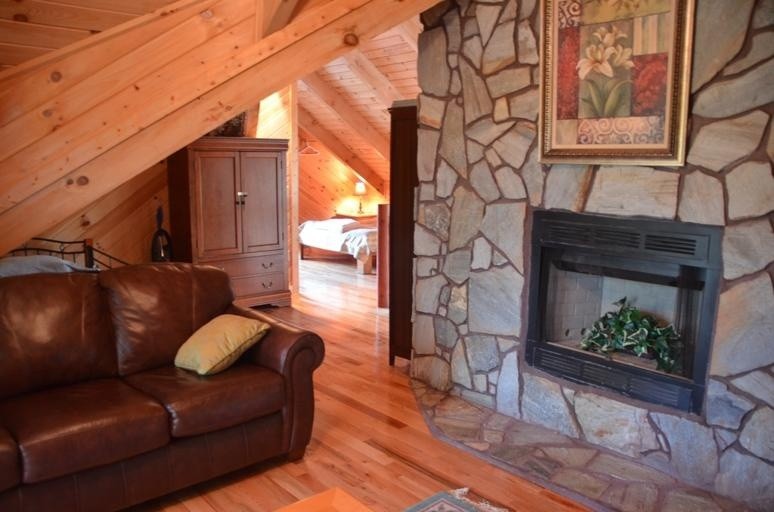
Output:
[{"xmin": 403, "ymin": 486, "xmax": 499, "ymax": 511}]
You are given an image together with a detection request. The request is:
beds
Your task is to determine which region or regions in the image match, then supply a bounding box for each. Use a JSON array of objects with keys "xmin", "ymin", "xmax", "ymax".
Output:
[{"xmin": 299, "ymin": 218, "xmax": 378, "ymax": 275}]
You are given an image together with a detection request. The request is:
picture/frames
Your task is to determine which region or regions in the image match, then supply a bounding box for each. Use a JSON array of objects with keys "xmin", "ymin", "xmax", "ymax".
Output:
[{"xmin": 537, "ymin": 1, "xmax": 696, "ymax": 168}]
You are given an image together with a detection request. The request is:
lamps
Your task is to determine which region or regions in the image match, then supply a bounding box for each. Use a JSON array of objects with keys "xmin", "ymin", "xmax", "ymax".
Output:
[{"xmin": 355, "ymin": 182, "xmax": 366, "ymax": 214}]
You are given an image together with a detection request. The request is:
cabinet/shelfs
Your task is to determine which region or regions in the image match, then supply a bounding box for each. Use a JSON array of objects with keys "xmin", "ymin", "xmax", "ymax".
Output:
[{"xmin": 167, "ymin": 136, "xmax": 292, "ymax": 310}]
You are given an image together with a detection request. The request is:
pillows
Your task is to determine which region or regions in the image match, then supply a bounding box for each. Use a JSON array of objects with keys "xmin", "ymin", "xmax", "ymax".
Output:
[
  {"xmin": 173, "ymin": 312, "xmax": 272, "ymax": 376},
  {"xmin": 0, "ymin": 270, "xmax": 119, "ymax": 404},
  {"xmin": 99, "ymin": 262, "xmax": 236, "ymax": 376}
]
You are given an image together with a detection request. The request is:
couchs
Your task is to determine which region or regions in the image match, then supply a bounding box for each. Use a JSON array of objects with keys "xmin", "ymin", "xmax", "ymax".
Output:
[{"xmin": 0, "ymin": 303, "xmax": 325, "ymax": 511}]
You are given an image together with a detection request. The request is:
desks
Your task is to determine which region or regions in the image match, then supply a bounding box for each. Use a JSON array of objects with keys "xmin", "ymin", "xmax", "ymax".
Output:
[{"xmin": 335, "ymin": 212, "xmax": 378, "ymax": 227}]
